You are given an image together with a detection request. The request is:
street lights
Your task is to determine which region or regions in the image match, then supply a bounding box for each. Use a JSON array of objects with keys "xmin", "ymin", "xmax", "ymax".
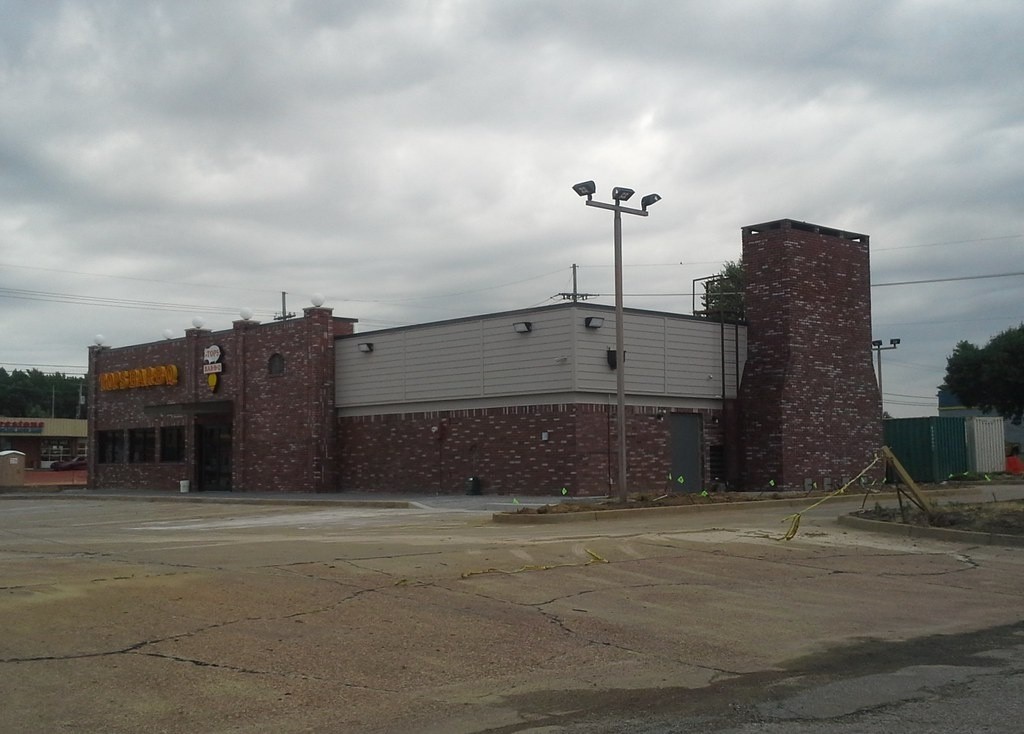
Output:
[
  {"xmin": 872, "ymin": 339, "xmax": 901, "ymax": 398},
  {"xmin": 573, "ymin": 180, "xmax": 663, "ymax": 504}
]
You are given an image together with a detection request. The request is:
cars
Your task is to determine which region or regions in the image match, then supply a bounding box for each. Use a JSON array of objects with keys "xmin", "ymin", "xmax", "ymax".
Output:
[{"xmin": 51, "ymin": 455, "xmax": 88, "ymax": 470}]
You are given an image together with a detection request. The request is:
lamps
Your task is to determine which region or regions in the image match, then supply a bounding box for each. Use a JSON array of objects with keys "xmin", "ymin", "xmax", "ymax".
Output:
[
  {"xmin": 513, "ymin": 322, "xmax": 532, "ymax": 333},
  {"xmin": 358, "ymin": 343, "xmax": 374, "ymax": 353},
  {"xmin": 584, "ymin": 317, "xmax": 604, "ymax": 329}
]
